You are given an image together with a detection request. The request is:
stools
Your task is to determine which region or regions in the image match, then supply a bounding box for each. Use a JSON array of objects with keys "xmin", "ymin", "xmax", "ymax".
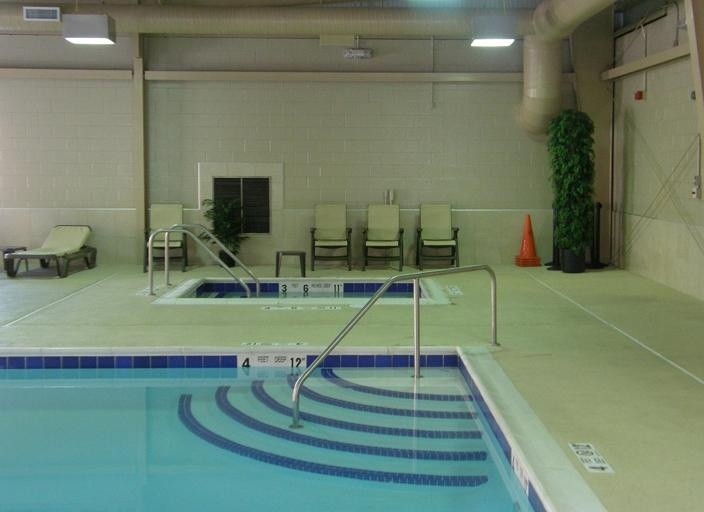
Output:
[
  {"xmin": 274, "ymin": 250, "xmax": 306, "ymax": 277},
  {"xmin": 0, "ymin": 245, "xmax": 29, "ymax": 273}
]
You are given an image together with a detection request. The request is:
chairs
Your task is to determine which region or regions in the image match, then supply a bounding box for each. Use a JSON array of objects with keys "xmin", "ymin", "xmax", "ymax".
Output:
[
  {"xmin": 361, "ymin": 205, "xmax": 404, "ymax": 271},
  {"xmin": 309, "ymin": 204, "xmax": 353, "ymax": 272},
  {"xmin": 142, "ymin": 203, "xmax": 189, "ymax": 273},
  {"xmin": 414, "ymin": 203, "xmax": 460, "ymax": 272},
  {"xmin": 4, "ymin": 224, "xmax": 98, "ymax": 279}
]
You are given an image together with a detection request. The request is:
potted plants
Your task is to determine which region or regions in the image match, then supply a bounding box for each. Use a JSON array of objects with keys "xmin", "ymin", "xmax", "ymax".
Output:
[
  {"xmin": 548, "ymin": 107, "xmax": 596, "ymax": 275},
  {"xmin": 195, "ymin": 197, "xmax": 254, "ymax": 269}
]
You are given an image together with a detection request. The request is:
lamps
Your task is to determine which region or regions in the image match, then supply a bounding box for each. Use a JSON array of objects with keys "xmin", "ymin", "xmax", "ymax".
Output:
[
  {"xmin": 61, "ymin": 0, "xmax": 117, "ymax": 45},
  {"xmin": 469, "ymin": 0, "xmax": 517, "ymax": 48}
]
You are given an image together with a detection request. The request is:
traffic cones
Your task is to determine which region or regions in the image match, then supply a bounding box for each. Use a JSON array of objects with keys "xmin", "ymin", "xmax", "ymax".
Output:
[{"xmin": 514, "ymin": 214, "xmax": 542, "ymax": 267}]
